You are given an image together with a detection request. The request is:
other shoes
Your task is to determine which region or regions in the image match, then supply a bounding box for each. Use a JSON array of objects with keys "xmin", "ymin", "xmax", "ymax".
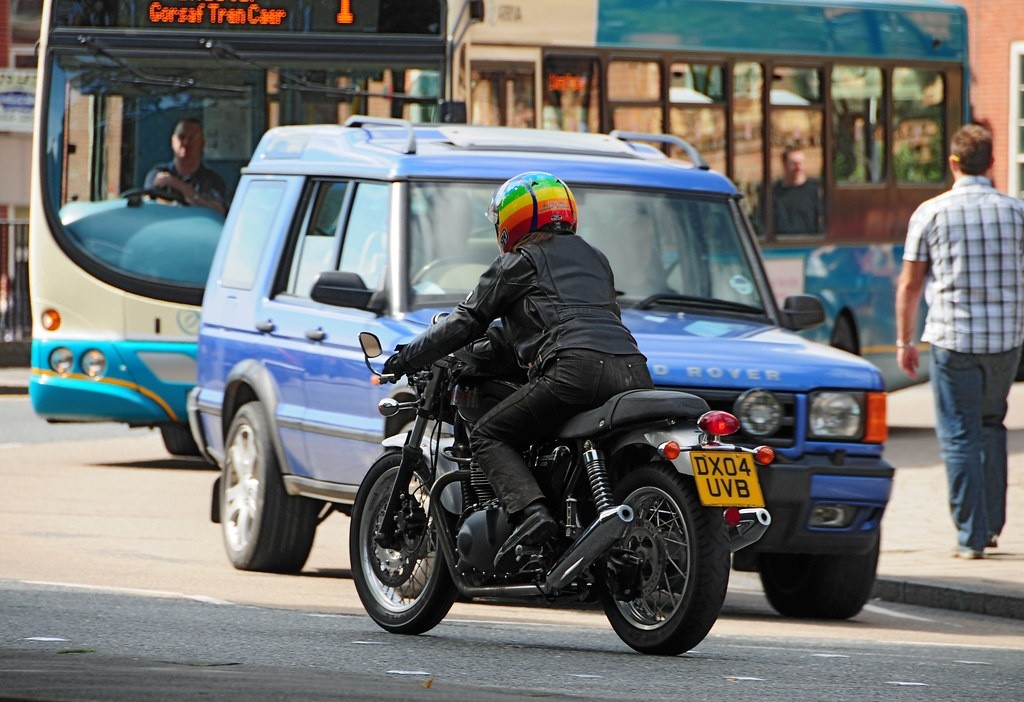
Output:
[
  {"xmin": 985, "ymin": 538, "xmax": 997, "ymax": 547},
  {"xmin": 494, "ymin": 504, "xmax": 556, "ymax": 568},
  {"xmin": 950, "ymin": 542, "xmax": 984, "ymax": 558}
]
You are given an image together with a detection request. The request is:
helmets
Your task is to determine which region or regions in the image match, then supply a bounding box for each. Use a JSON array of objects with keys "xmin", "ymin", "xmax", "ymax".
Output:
[{"xmin": 485, "ymin": 171, "xmax": 577, "ymax": 252}]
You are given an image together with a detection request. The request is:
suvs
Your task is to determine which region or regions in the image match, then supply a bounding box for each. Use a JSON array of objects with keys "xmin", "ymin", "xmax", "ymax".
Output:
[{"xmin": 187, "ymin": 116, "xmax": 898, "ymax": 623}]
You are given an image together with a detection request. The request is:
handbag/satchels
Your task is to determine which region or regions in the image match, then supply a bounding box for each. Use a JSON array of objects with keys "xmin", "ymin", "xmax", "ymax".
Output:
[{"xmin": 455, "ymin": 318, "xmax": 531, "ymax": 383}]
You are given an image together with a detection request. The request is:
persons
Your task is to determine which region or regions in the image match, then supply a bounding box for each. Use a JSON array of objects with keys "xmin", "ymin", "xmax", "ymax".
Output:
[
  {"xmin": 144, "ymin": 118, "xmax": 227, "ymax": 213},
  {"xmin": 896, "ymin": 125, "xmax": 1024, "ymax": 558},
  {"xmin": 772, "ymin": 148, "xmax": 824, "ymax": 235},
  {"xmin": 379, "ymin": 172, "xmax": 653, "ymax": 566}
]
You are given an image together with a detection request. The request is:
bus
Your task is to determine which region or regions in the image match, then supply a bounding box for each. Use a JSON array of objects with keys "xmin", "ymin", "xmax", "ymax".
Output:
[{"xmin": 28, "ymin": 1, "xmax": 975, "ymax": 455}]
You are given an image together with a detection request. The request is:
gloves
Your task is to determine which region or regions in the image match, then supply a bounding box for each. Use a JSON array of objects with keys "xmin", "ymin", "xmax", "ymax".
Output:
[{"xmin": 380, "ymin": 353, "xmax": 405, "ymax": 384}]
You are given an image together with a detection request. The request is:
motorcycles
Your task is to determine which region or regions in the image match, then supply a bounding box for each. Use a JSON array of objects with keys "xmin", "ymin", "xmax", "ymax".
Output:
[{"xmin": 348, "ymin": 310, "xmax": 771, "ymax": 657}]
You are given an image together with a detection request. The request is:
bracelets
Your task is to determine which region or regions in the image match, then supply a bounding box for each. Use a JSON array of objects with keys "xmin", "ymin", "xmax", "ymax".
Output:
[{"xmin": 896, "ymin": 339, "xmax": 913, "ymax": 348}]
